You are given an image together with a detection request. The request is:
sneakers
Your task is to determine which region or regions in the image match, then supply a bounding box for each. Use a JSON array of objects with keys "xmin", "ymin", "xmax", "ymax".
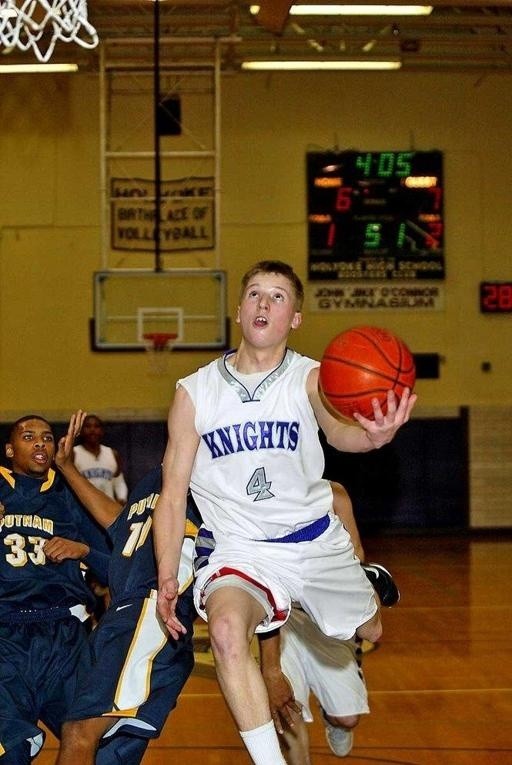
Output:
[
  {"xmin": 362, "ymin": 563, "xmax": 400, "ymax": 608},
  {"xmin": 317, "ymin": 704, "xmax": 354, "ymax": 758}
]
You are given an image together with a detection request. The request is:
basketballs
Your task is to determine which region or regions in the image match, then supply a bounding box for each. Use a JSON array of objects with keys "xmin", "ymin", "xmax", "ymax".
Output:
[{"xmin": 320, "ymin": 325, "xmax": 417, "ymax": 422}]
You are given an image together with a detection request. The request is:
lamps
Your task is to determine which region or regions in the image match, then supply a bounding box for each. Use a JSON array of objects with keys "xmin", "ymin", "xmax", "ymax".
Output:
[
  {"xmin": 234, "ymin": 55, "xmax": 405, "ymax": 73},
  {"xmin": 0, "ymin": 60, "xmax": 85, "ymax": 76},
  {"xmin": 290, "ymin": 1, "xmax": 434, "ymax": 20}
]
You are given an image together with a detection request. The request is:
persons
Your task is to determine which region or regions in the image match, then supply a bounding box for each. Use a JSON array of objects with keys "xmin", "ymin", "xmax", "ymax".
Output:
[
  {"xmin": 1, "ymin": 409, "xmax": 370, "ymax": 764},
  {"xmin": 152, "ymin": 259, "xmax": 420, "ymax": 765}
]
